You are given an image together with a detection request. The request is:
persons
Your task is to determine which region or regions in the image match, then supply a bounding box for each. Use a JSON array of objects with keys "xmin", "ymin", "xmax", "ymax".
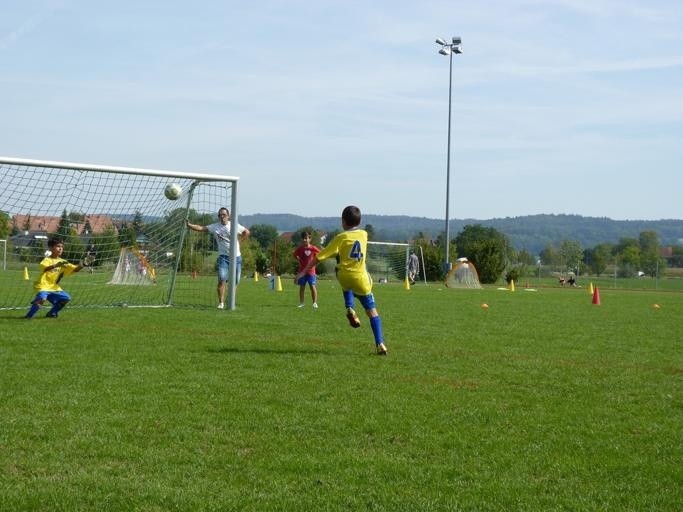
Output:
[
  {"xmin": 309, "ymin": 206, "xmax": 387, "ymax": 356},
  {"xmin": 182, "ymin": 208, "xmax": 250, "ymax": 309},
  {"xmin": 293, "ymin": 232, "xmax": 321, "ymax": 309},
  {"xmin": 80, "ymin": 238, "xmax": 97, "ymax": 273},
  {"xmin": 25, "ymin": 238, "xmax": 95, "ymax": 318},
  {"xmin": 568, "ymin": 276, "xmax": 576, "ymax": 288},
  {"xmin": 404, "ymin": 249, "xmax": 420, "ymax": 285}
]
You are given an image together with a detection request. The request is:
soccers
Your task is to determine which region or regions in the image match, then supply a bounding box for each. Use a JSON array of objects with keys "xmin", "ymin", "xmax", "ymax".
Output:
[{"xmin": 164, "ymin": 183, "xmax": 182, "ymax": 200}]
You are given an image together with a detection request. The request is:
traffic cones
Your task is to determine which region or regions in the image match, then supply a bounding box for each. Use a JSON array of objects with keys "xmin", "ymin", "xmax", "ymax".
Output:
[
  {"xmin": 192, "ymin": 271, "xmax": 196, "ymax": 279},
  {"xmin": 24, "ymin": 266, "xmax": 30, "ymax": 280},
  {"xmin": 276, "ymin": 276, "xmax": 282, "ymax": 291},
  {"xmin": 525, "ymin": 282, "xmax": 530, "ymax": 288},
  {"xmin": 509, "ymin": 280, "xmax": 516, "ymax": 291},
  {"xmin": 590, "ymin": 283, "xmax": 594, "ymax": 294},
  {"xmin": 254, "ymin": 272, "xmax": 259, "ymax": 281},
  {"xmin": 592, "ymin": 287, "xmax": 600, "ymax": 305},
  {"xmin": 404, "ymin": 274, "xmax": 410, "ymax": 289}
]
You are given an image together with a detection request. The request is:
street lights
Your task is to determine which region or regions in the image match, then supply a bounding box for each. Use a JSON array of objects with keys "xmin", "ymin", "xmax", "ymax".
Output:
[{"xmin": 436, "ymin": 35, "xmax": 462, "ymax": 263}]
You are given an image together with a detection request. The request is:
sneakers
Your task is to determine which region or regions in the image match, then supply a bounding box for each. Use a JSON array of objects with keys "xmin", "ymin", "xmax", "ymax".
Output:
[
  {"xmin": 345, "ymin": 307, "xmax": 360, "ymax": 328},
  {"xmin": 292, "ymin": 302, "xmax": 318, "ymax": 306},
  {"xmin": 376, "ymin": 342, "xmax": 388, "ymax": 355},
  {"xmin": 217, "ymin": 303, "xmax": 224, "ymax": 309}
]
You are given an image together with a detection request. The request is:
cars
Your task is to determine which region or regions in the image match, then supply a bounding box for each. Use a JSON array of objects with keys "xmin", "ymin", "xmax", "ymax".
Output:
[
  {"xmin": 10, "ymin": 230, "xmax": 102, "ymax": 265},
  {"xmin": 108, "ymin": 231, "xmax": 185, "ymax": 272}
]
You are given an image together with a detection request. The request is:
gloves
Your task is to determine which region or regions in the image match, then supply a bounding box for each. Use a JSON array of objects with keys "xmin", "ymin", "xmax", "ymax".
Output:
[
  {"xmin": 81, "ymin": 255, "xmax": 96, "ymax": 268},
  {"xmin": 53, "ymin": 261, "xmax": 68, "ymax": 268}
]
